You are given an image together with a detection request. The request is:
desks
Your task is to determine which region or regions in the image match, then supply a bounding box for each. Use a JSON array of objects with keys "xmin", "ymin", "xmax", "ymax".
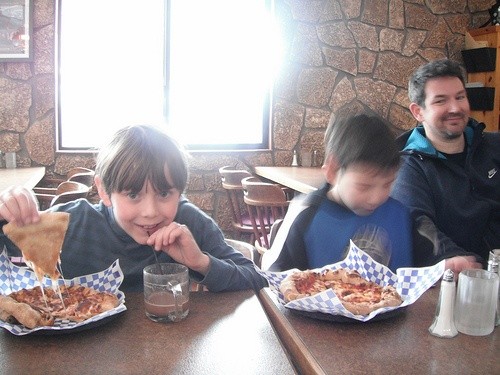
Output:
[
  {"xmin": 259, "ymin": 281, "xmax": 500, "ymax": 375},
  {"xmin": 0, "ymin": 290, "xmax": 299, "ymax": 375},
  {"xmin": 0, "ymin": 167, "xmax": 46, "ymax": 194},
  {"xmin": 254, "ymin": 165, "xmax": 324, "ymax": 196}
]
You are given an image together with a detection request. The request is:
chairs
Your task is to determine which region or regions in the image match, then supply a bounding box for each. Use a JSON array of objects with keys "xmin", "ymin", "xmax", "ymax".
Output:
[
  {"xmin": 219, "ymin": 166, "xmax": 293, "ymax": 264},
  {"xmin": 33, "ymin": 167, "xmax": 95, "ymax": 207}
]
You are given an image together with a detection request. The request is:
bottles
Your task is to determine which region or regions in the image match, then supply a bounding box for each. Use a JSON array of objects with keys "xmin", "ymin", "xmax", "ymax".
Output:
[
  {"xmin": 426, "ymin": 268, "xmax": 459, "ymax": 339},
  {"xmin": 488, "ymin": 249, "xmax": 500, "ymax": 327},
  {"xmin": 290, "ymin": 149, "xmax": 299, "ymax": 167},
  {"xmin": 312, "ymin": 150, "xmax": 319, "ymax": 167},
  {"xmin": 0, "ymin": 150, "xmax": 3, "ymax": 168}
]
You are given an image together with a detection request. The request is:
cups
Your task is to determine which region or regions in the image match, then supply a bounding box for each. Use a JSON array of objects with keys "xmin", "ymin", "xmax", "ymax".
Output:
[
  {"xmin": 453, "ymin": 268, "xmax": 500, "ymax": 337},
  {"xmin": 143, "ymin": 262, "xmax": 190, "ymax": 322},
  {"xmin": 299, "ymin": 148, "xmax": 312, "ymax": 167},
  {"xmin": 6, "ymin": 152, "xmax": 17, "ymax": 168}
]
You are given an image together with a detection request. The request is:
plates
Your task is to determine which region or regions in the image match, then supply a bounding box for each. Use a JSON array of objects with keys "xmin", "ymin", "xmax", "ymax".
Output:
[
  {"xmin": 291, "ymin": 308, "xmax": 407, "ymax": 324},
  {"xmin": 0, "ymin": 311, "xmax": 128, "ymax": 334}
]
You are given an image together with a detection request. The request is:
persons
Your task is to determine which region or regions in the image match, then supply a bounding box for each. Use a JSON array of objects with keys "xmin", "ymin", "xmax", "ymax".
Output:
[
  {"xmin": 263, "ymin": 114, "xmax": 484, "ymax": 288},
  {"xmin": 389, "ymin": 59, "xmax": 500, "ymax": 273},
  {"xmin": 0, "ymin": 122, "xmax": 269, "ymax": 292}
]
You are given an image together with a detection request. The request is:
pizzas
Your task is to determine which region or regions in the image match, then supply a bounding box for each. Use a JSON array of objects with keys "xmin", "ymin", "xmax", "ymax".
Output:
[
  {"xmin": 0, "ymin": 283, "xmax": 120, "ymax": 329},
  {"xmin": 3, "ymin": 210, "xmax": 70, "ymax": 284},
  {"xmin": 279, "ymin": 267, "xmax": 403, "ymax": 316}
]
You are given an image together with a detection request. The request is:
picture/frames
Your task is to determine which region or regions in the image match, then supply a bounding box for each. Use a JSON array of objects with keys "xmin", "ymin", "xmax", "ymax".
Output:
[{"xmin": 0, "ymin": 0, "xmax": 33, "ymax": 63}]
